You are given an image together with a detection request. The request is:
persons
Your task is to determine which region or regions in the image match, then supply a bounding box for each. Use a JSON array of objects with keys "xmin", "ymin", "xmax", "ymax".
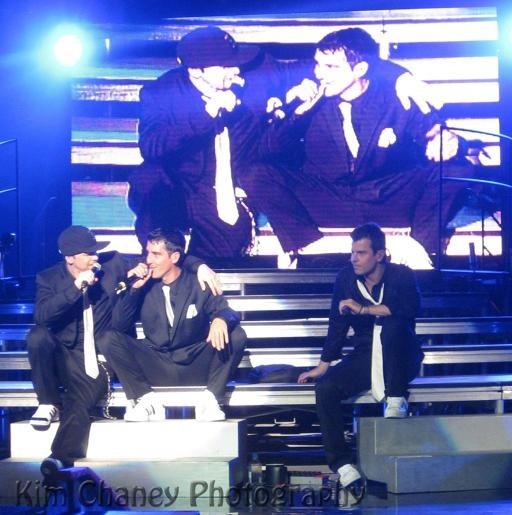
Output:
[
  {"xmin": 238, "ymin": 27, "xmax": 475, "ymax": 274},
  {"xmin": 297, "ymin": 221, "xmax": 424, "ymax": 488},
  {"xmin": 96, "ymin": 227, "xmax": 248, "ymax": 422},
  {"xmin": 127, "ymin": 27, "xmax": 447, "ymax": 264},
  {"xmin": 25, "ymin": 224, "xmax": 225, "ymax": 487}
]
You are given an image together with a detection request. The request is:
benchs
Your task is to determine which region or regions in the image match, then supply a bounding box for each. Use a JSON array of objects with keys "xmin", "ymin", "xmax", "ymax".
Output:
[
  {"xmin": 75, "ymin": 9, "xmax": 502, "ymax": 254},
  {"xmin": 0, "ymin": 262, "xmax": 512, "ymax": 405}
]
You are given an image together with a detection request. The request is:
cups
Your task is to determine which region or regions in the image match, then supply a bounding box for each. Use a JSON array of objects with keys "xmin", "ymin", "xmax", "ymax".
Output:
[{"xmin": 266, "ymin": 463, "xmax": 289, "ymax": 489}]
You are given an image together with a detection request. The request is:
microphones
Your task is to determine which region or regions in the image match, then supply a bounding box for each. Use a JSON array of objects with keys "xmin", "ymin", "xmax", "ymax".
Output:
[
  {"xmin": 274, "ymin": 85, "xmax": 318, "ymax": 120},
  {"xmin": 80, "ymin": 263, "xmax": 102, "ymax": 291},
  {"xmin": 114, "ymin": 268, "xmax": 148, "ymax": 294},
  {"xmin": 341, "ymin": 294, "xmax": 361, "ymax": 319},
  {"xmin": 216, "ymin": 76, "xmax": 246, "ymax": 120}
]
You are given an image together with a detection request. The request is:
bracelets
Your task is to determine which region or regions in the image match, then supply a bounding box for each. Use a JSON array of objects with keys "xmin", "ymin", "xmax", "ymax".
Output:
[{"xmin": 357, "ymin": 304, "xmax": 369, "ymax": 315}]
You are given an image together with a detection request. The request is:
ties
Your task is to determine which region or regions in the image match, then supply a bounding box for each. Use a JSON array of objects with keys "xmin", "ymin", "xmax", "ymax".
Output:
[
  {"xmin": 335, "ymin": 101, "xmax": 364, "ymax": 160},
  {"xmin": 80, "ymin": 284, "xmax": 100, "ymax": 380},
  {"xmin": 161, "ymin": 285, "xmax": 174, "ymax": 330},
  {"xmin": 213, "ymin": 122, "xmax": 240, "ymax": 227},
  {"xmin": 355, "ymin": 278, "xmax": 388, "ymax": 403}
]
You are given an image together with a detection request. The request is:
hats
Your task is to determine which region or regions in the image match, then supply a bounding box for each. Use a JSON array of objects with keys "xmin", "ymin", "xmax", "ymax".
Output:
[
  {"xmin": 57, "ymin": 225, "xmax": 111, "ymax": 257},
  {"xmin": 175, "ymin": 26, "xmax": 261, "ymax": 70}
]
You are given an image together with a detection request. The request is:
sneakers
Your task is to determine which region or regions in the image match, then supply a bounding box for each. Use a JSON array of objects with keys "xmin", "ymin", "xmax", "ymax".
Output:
[
  {"xmin": 123, "ymin": 401, "xmax": 166, "ymax": 421},
  {"xmin": 39, "ymin": 457, "xmax": 66, "ymax": 490},
  {"xmin": 30, "ymin": 402, "xmax": 62, "ymax": 430},
  {"xmin": 191, "ymin": 397, "xmax": 227, "ymax": 421},
  {"xmin": 335, "ymin": 461, "xmax": 362, "ymax": 489},
  {"xmin": 383, "ymin": 395, "xmax": 411, "ymax": 420}
]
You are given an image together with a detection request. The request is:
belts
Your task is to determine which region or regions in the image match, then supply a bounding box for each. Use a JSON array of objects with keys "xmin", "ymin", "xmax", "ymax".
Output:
[
  {"xmin": 233, "ymin": 185, "xmax": 250, "ymax": 201},
  {"xmin": 94, "ymin": 353, "xmax": 110, "ymax": 365}
]
